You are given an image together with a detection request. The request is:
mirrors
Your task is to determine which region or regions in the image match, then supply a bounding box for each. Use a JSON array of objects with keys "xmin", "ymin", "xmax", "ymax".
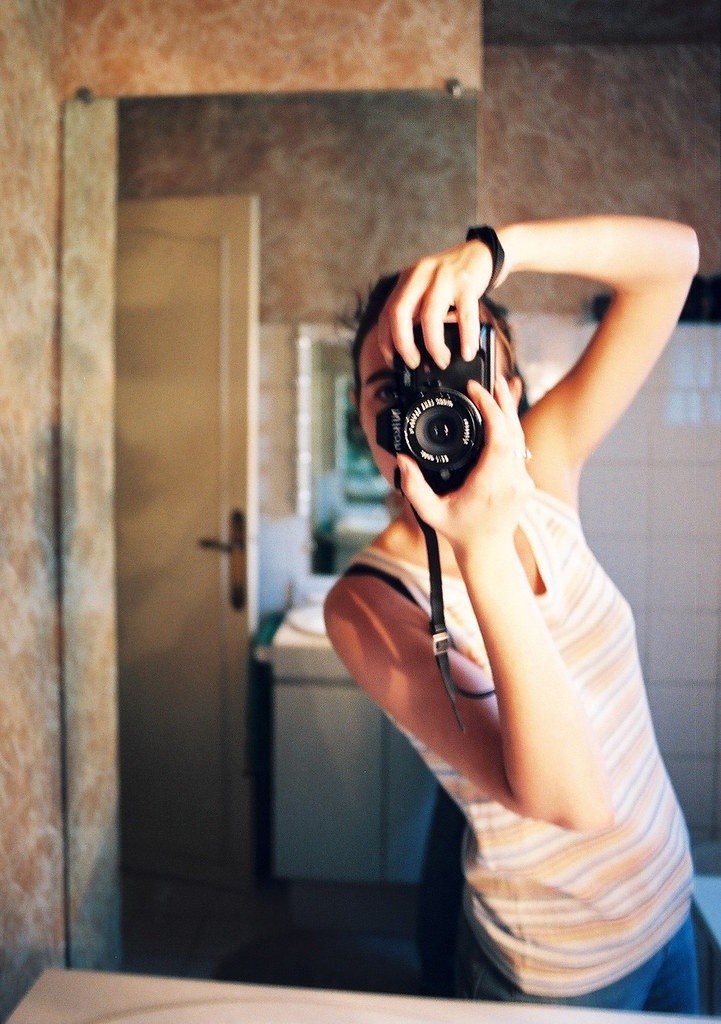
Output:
[{"xmin": 63, "ymin": 84, "xmax": 721, "ymax": 1022}]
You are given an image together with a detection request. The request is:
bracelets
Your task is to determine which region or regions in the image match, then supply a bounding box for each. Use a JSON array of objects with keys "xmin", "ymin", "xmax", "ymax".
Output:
[{"xmin": 466, "ymin": 225, "xmax": 504, "ymax": 299}]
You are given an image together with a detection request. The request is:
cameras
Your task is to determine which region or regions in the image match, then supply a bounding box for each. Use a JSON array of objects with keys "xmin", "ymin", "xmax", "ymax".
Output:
[{"xmin": 374, "ymin": 321, "xmax": 494, "ymax": 498}]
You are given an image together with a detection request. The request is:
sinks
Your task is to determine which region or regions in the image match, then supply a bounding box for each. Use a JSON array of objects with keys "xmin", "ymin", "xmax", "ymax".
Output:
[{"xmin": 286, "ymin": 604, "xmax": 330, "ymax": 637}]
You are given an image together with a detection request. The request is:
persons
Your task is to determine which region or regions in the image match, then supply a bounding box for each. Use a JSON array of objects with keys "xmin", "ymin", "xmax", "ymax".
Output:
[{"xmin": 324, "ymin": 213, "xmax": 701, "ymax": 1016}]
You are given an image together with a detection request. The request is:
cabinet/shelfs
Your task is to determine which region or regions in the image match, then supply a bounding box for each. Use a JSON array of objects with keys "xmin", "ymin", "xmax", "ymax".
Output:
[{"xmin": 271, "ymin": 653, "xmax": 439, "ymax": 883}]
